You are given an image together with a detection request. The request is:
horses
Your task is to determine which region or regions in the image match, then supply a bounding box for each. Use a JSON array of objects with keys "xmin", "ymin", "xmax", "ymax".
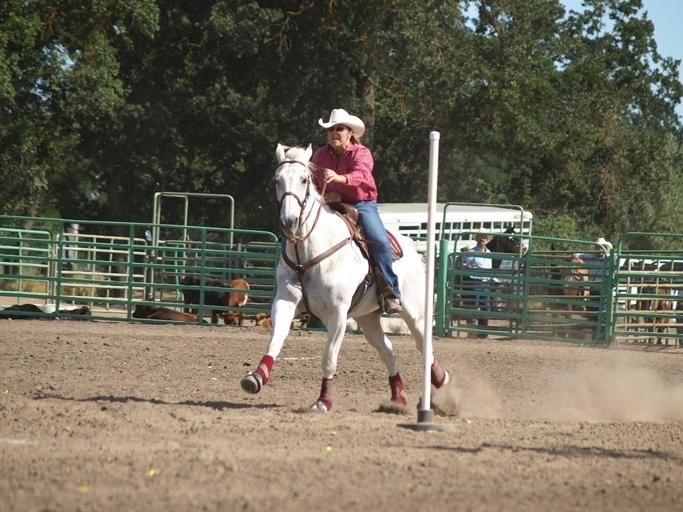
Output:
[
  {"xmin": 549, "ymin": 244, "xmax": 590, "ymax": 338},
  {"xmin": 241, "ymin": 142, "xmax": 450, "ymax": 413},
  {"xmin": 637, "ymin": 278, "xmax": 673, "ymax": 344},
  {"xmin": 433, "ymin": 223, "xmax": 526, "ymax": 338}
]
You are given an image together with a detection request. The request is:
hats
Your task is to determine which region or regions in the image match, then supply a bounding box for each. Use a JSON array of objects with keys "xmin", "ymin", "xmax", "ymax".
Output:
[
  {"xmin": 591, "ymin": 237, "xmax": 613, "ymax": 250},
  {"xmin": 318, "ymin": 108, "xmax": 365, "ymax": 139},
  {"xmin": 473, "ymin": 229, "xmax": 493, "ymax": 241}
]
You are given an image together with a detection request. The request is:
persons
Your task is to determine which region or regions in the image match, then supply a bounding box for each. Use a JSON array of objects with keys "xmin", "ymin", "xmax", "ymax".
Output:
[
  {"xmin": 573, "ymin": 238, "xmax": 616, "ymax": 346},
  {"xmin": 310, "ymin": 108, "xmax": 404, "ymax": 314},
  {"xmin": 461, "ymin": 227, "xmax": 502, "ymax": 338}
]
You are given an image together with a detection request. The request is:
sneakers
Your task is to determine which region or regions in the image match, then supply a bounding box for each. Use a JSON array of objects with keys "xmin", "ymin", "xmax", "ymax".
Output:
[{"xmin": 385, "ymin": 296, "xmax": 402, "ymax": 314}]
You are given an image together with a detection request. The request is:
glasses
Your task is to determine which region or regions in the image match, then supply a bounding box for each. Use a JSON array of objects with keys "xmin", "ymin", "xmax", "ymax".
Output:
[{"xmin": 326, "ymin": 127, "xmax": 349, "ymax": 131}]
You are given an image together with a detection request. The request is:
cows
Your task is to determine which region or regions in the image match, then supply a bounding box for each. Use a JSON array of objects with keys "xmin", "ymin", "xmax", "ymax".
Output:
[
  {"xmin": 132, "ymin": 305, "xmax": 208, "ymax": 323},
  {"xmin": 222, "ymin": 278, "xmax": 249, "ymax": 327},
  {"xmin": 179, "ymin": 276, "xmax": 229, "ymax": 323}
]
